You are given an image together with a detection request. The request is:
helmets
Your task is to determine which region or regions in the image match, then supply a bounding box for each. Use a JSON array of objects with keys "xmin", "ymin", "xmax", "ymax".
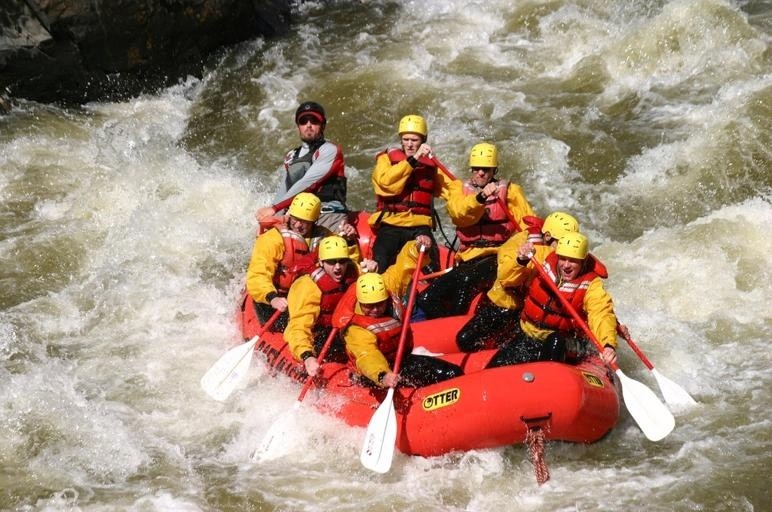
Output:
[
  {"xmin": 542, "ymin": 212, "xmax": 580, "ymax": 240},
  {"xmin": 288, "ymin": 192, "xmax": 323, "ymax": 221},
  {"xmin": 356, "ymin": 271, "xmax": 389, "ymax": 305},
  {"xmin": 317, "ymin": 236, "xmax": 351, "ymax": 261},
  {"xmin": 557, "ymin": 232, "xmax": 589, "ymax": 260},
  {"xmin": 470, "ymin": 143, "xmax": 500, "ymax": 169},
  {"xmin": 399, "ymin": 115, "xmax": 428, "ymax": 136}
]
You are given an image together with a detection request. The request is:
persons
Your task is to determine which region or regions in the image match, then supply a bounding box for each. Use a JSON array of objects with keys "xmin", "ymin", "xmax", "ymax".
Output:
[
  {"xmin": 457, "ymin": 212, "xmax": 630, "ymax": 353},
  {"xmin": 485, "ymin": 232, "xmax": 618, "ymax": 369},
  {"xmin": 345, "ymin": 235, "xmax": 464, "ymax": 388},
  {"xmin": 245, "ymin": 192, "xmax": 360, "ymax": 332},
  {"xmin": 368, "ymin": 115, "xmax": 453, "ymax": 283},
  {"xmin": 418, "ymin": 144, "xmax": 536, "ymax": 318},
  {"xmin": 254, "ymin": 102, "xmax": 350, "ymax": 233},
  {"xmin": 283, "ymin": 236, "xmax": 379, "ymax": 377}
]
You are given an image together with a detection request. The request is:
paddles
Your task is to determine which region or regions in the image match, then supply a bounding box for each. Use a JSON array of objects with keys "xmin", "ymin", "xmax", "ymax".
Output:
[
  {"xmin": 525, "ymin": 251, "xmax": 696, "ymax": 442},
  {"xmin": 199, "ymin": 310, "xmax": 280, "ymax": 403},
  {"xmin": 360, "ymin": 244, "xmax": 427, "ymax": 474},
  {"xmin": 249, "ymin": 328, "xmax": 335, "ymax": 460}
]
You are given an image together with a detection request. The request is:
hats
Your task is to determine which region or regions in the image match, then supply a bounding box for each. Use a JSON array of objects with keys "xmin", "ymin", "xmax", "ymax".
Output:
[{"xmin": 294, "ymin": 102, "xmax": 327, "ymax": 122}]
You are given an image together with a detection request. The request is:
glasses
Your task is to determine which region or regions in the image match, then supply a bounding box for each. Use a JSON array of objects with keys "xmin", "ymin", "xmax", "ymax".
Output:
[
  {"xmin": 324, "ymin": 259, "xmax": 348, "ymax": 265},
  {"xmin": 298, "ymin": 117, "xmax": 321, "ymax": 126}
]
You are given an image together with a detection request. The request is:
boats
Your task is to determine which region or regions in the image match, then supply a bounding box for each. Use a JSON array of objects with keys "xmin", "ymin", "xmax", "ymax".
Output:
[{"xmin": 237, "ymin": 210, "xmax": 622, "ymax": 457}]
